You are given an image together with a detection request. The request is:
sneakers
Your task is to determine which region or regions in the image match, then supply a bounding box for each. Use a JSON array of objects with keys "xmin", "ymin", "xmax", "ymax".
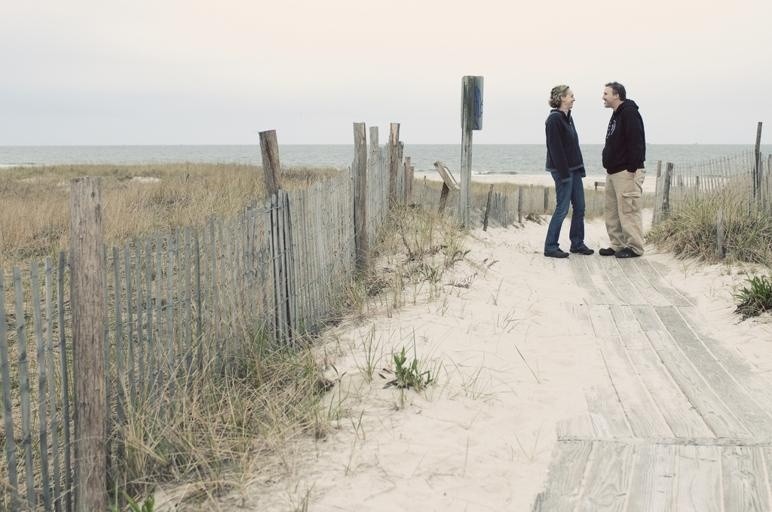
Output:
[
  {"xmin": 570, "ymin": 244, "xmax": 594, "ymax": 255},
  {"xmin": 616, "ymin": 249, "xmax": 640, "ymax": 257},
  {"xmin": 544, "ymin": 248, "xmax": 569, "ymax": 258},
  {"xmin": 599, "ymin": 248, "xmax": 616, "ymax": 256}
]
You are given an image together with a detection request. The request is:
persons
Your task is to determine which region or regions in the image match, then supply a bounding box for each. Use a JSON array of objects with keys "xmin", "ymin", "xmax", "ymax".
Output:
[
  {"xmin": 599, "ymin": 82, "xmax": 648, "ymax": 260},
  {"xmin": 543, "ymin": 83, "xmax": 596, "ymax": 259}
]
